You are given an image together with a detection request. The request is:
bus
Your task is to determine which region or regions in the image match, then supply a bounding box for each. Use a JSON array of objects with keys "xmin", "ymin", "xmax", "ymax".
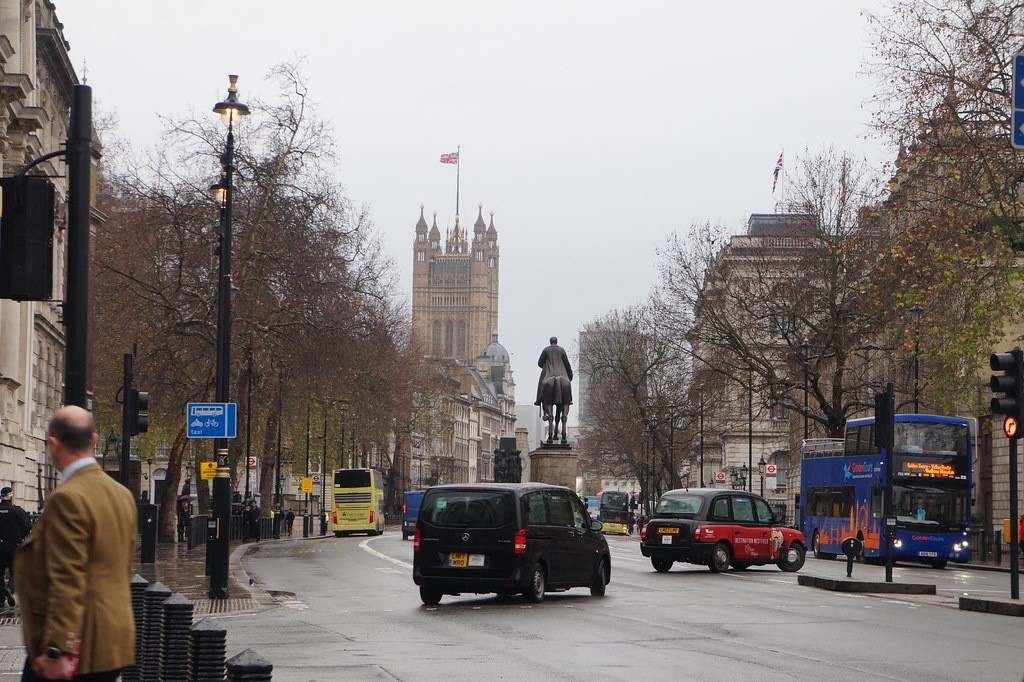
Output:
[
  {"xmin": 800, "ymin": 412, "xmax": 977, "ymax": 569},
  {"xmin": 597, "ymin": 491, "xmax": 633, "ymax": 536},
  {"xmin": 330, "ymin": 467, "xmax": 388, "ymax": 536}
]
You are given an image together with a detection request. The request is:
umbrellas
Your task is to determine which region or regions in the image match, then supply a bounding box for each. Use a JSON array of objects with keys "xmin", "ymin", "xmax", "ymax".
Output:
[{"xmin": 179, "ymin": 494, "xmax": 195, "ymax": 501}]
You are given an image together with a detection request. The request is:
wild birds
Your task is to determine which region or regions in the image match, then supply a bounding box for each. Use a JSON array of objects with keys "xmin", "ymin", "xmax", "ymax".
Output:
[{"xmin": 249, "ymin": 578, "xmax": 254, "ymax": 586}]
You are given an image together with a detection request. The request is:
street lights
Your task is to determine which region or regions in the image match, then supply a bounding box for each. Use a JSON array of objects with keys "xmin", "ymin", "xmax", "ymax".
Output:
[
  {"xmin": 275, "ymin": 358, "xmax": 286, "ymax": 512},
  {"xmin": 645, "ymin": 401, "xmax": 675, "ymax": 515},
  {"xmin": 757, "ymin": 454, "xmax": 767, "ymax": 497},
  {"xmin": 798, "ymin": 336, "xmax": 813, "ymax": 438},
  {"xmin": 739, "ymin": 462, "xmax": 748, "ymax": 491},
  {"xmin": 205, "ymin": 75, "xmax": 252, "ymax": 601},
  {"xmin": 695, "ymin": 383, "xmax": 707, "ymax": 487},
  {"xmin": 185, "ymin": 459, "xmax": 193, "ymax": 513},
  {"xmin": 908, "ymin": 302, "xmax": 924, "ymax": 414},
  {"xmin": 303, "ymin": 392, "xmax": 357, "ymax": 538},
  {"xmin": 729, "ymin": 467, "xmax": 739, "ymax": 489},
  {"xmin": 738, "ymin": 361, "xmax": 752, "ymax": 491}
]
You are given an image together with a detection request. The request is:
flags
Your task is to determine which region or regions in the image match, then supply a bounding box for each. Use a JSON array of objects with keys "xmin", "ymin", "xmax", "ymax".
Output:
[
  {"xmin": 440, "ymin": 152, "xmax": 458, "ymax": 164},
  {"xmin": 772, "ymin": 153, "xmax": 782, "ymax": 192}
]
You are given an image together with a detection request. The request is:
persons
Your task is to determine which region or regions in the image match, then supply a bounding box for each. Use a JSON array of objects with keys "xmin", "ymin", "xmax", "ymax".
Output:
[
  {"xmin": 0, "ymin": 487, "xmax": 21, "ymax": 608},
  {"xmin": 629, "ymin": 516, "xmax": 644, "ymax": 534},
  {"xmin": 533, "ymin": 337, "xmax": 574, "ymax": 406},
  {"xmin": 245, "ymin": 500, "xmax": 261, "ymax": 542},
  {"xmin": 14, "ymin": 404, "xmax": 136, "ymax": 682},
  {"xmin": 286, "ymin": 508, "xmax": 295, "ymax": 536},
  {"xmin": 178, "ymin": 501, "xmax": 187, "ymax": 542}
]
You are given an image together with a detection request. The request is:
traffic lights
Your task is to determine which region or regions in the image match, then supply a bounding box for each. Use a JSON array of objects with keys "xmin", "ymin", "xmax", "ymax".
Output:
[
  {"xmin": 637, "ymin": 493, "xmax": 643, "ymax": 504},
  {"xmin": 585, "ymin": 498, "xmax": 588, "ymax": 510},
  {"xmin": 1003, "ymin": 415, "xmax": 1018, "ymax": 439},
  {"xmin": 629, "ymin": 497, "xmax": 634, "ymax": 508},
  {"xmin": 133, "ymin": 387, "xmax": 151, "ymax": 435},
  {"xmin": 988, "ymin": 353, "xmax": 1015, "ymax": 413}
]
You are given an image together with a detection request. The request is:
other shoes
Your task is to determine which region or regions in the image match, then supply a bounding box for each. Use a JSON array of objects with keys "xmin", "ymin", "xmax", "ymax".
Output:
[
  {"xmin": 0, "ymin": 602, "xmax": 5, "ymax": 607},
  {"xmin": 4, "ymin": 588, "xmax": 16, "ymax": 607}
]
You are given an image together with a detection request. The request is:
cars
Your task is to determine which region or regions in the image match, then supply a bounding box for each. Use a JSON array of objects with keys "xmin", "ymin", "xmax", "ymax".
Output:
[{"xmin": 585, "ymin": 495, "xmax": 600, "ymax": 508}]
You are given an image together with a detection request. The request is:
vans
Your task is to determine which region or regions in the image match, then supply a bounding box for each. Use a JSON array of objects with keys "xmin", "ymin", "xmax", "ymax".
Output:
[
  {"xmin": 412, "ymin": 481, "xmax": 612, "ymax": 604},
  {"xmin": 401, "ymin": 490, "xmax": 428, "ymax": 540}
]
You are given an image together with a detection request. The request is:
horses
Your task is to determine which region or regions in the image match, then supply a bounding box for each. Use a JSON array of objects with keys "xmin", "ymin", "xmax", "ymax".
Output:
[{"xmin": 542, "ymin": 376, "xmax": 572, "ymax": 443}]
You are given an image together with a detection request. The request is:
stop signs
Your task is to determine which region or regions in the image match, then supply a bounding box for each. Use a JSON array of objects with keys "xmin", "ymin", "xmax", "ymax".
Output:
[
  {"xmin": 718, "ymin": 473, "xmax": 724, "ymax": 479},
  {"xmin": 247, "ymin": 458, "xmax": 255, "ymax": 465},
  {"xmin": 314, "ymin": 476, "xmax": 319, "ymax": 481},
  {"xmin": 767, "ymin": 466, "xmax": 775, "ymax": 472}
]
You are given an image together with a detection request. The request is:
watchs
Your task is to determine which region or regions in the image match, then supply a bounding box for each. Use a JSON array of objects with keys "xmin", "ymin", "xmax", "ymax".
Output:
[{"xmin": 46, "ymin": 645, "xmax": 68, "ymax": 661}]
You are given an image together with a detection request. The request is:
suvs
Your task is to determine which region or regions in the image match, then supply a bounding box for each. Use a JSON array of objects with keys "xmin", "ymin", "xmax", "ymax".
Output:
[{"xmin": 640, "ymin": 488, "xmax": 809, "ymax": 574}]
